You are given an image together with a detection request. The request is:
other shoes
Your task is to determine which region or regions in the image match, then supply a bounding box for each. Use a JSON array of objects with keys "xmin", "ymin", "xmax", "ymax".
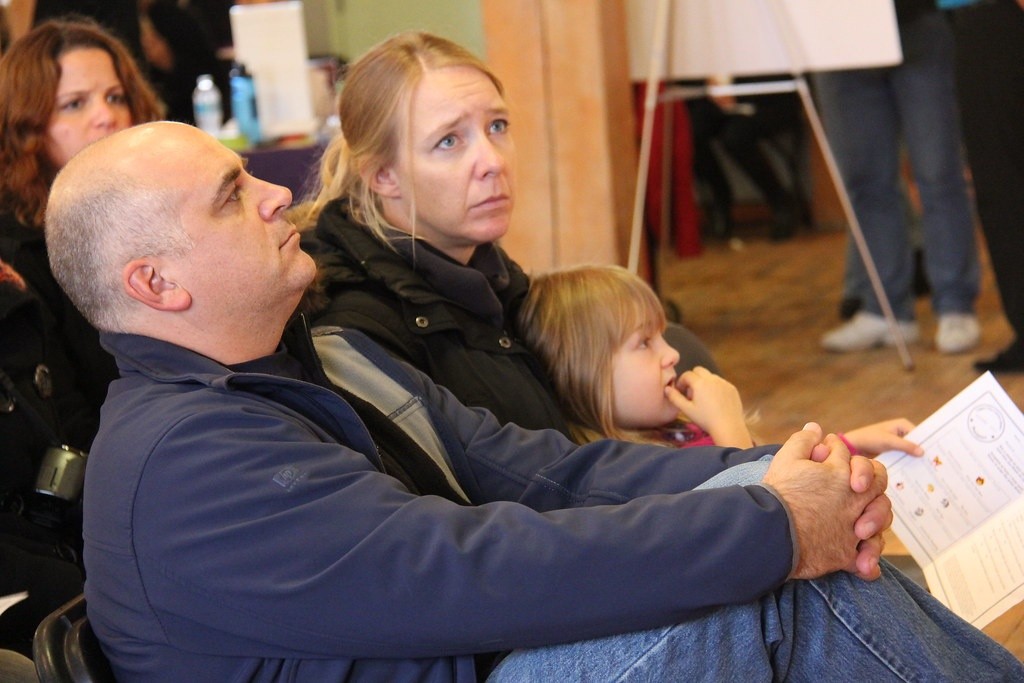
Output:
[{"xmin": 974, "ymin": 335, "xmax": 1024, "ymax": 375}]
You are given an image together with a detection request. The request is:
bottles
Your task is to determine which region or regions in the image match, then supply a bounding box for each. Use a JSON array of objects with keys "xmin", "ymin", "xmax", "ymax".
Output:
[
  {"xmin": 193, "ymin": 75, "xmax": 223, "ymax": 142},
  {"xmin": 230, "ymin": 66, "xmax": 261, "ymax": 145}
]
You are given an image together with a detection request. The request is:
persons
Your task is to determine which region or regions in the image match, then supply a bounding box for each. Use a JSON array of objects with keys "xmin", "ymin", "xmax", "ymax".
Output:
[
  {"xmin": 286, "ymin": 34, "xmax": 577, "ymax": 443},
  {"xmin": 44, "ymin": 119, "xmax": 1023, "ymax": 683},
  {"xmin": 935, "ymin": 0, "xmax": 1024, "ymax": 373},
  {"xmin": 675, "ymin": 72, "xmax": 814, "ymax": 243},
  {"xmin": 0, "ymin": 20, "xmax": 167, "ymax": 683},
  {"xmin": 811, "ymin": 0, "xmax": 983, "ymax": 354},
  {"xmin": 517, "ymin": 265, "xmax": 924, "ymax": 458}
]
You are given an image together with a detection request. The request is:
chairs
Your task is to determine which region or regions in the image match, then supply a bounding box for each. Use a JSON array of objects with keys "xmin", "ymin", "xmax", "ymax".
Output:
[{"xmin": 33, "ymin": 592, "xmax": 115, "ymax": 683}]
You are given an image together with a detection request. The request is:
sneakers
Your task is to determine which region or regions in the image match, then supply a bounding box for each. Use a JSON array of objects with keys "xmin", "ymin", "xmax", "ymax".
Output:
[
  {"xmin": 933, "ymin": 309, "xmax": 980, "ymax": 354},
  {"xmin": 819, "ymin": 310, "xmax": 918, "ymax": 352}
]
